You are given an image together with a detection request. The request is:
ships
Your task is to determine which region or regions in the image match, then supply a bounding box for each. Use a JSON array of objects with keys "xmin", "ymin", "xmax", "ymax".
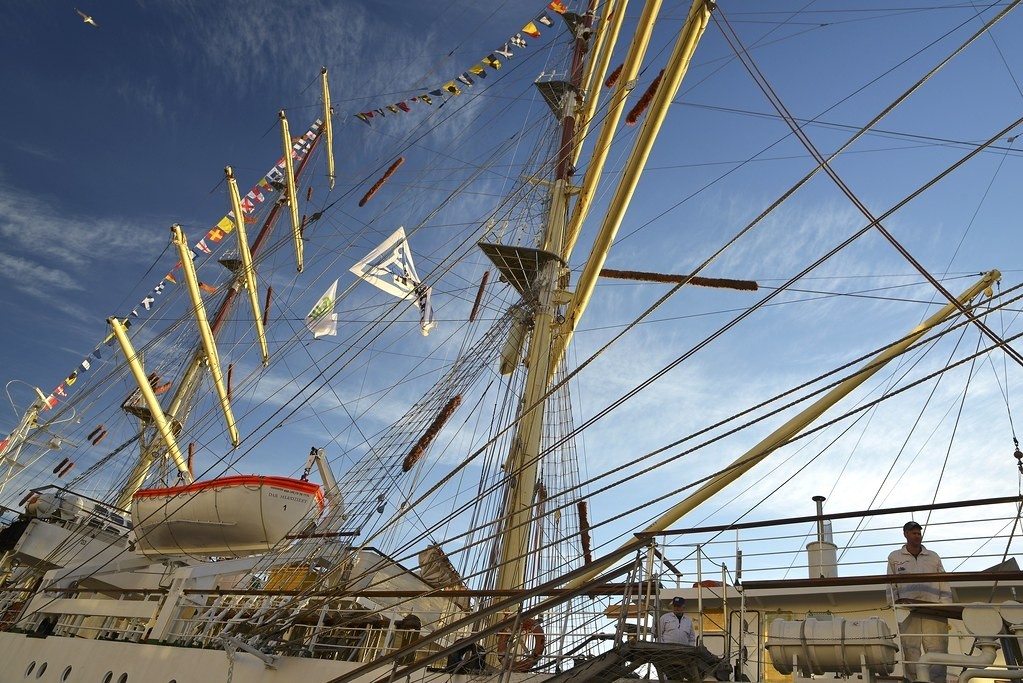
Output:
[{"xmin": 0, "ymin": 0, "xmax": 1023, "ymax": 682}]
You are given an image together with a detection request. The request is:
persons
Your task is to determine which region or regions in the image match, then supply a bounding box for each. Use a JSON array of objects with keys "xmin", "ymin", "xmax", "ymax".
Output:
[
  {"xmin": 651, "ymin": 597, "xmax": 695, "ymax": 646},
  {"xmin": 885, "ymin": 521, "xmax": 952, "ymax": 683}
]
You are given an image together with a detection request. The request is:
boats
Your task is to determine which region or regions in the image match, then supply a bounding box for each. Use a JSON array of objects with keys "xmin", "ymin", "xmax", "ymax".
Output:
[{"xmin": 130, "ymin": 473, "xmax": 326, "ymax": 558}]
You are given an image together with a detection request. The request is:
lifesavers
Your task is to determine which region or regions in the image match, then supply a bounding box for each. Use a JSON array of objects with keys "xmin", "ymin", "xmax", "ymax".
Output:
[
  {"xmin": 449, "ymin": 639, "xmax": 486, "ymax": 676},
  {"xmin": 496, "ymin": 618, "xmax": 546, "ymax": 672}
]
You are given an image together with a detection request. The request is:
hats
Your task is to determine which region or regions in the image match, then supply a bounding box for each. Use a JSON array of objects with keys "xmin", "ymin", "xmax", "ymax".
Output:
[
  {"xmin": 673, "ymin": 597, "xmax": 685, "ymax": 606},
  {"xmin": 903, "ymin": 521, "xmax": 922, "ymax": 530}
]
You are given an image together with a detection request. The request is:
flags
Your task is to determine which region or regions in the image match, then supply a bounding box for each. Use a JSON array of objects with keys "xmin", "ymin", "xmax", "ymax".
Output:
[
  {"xmin": 0, "ymin": 407, "xmax": 37, "ymax": 454},
  {"xmin": 429, "ymin": 89, "xmax": 447, "ymax": 101},
  {"xmin": 511, "ymin": 33, "xmax": 527, "ymax": 48},
  {"xmin": 482, "ymin": 54, "xmax": 501, "ymax": 70},
  {"xmin": 468, "ymin": 64, "xmax": 487, "ymax": 78},
  {"xmin": 386, "ymin": 105, "xmax": 399, "ymax": 113},
  {"xmin": 418, "ymin": 94, "xmax": 432, "ymax": 105},
  {"xmin": 456, "ymin": 72, "xmax": 474, "ymax": 87},
  {"xmin": 354, "ymin": 108, "xmax": 385, "ymax": 126},
  {"xmin": 292, "ymin": 119, "xmax": 323, "ymax": 161},
  {"xmin": 536, "ymin": 0, "xmax": 566, "ymax": 27},
  {"xmin": 189, "ymin": 209, "xmax": 236, "ymax": 261},
  {"xmin": 43, "ymin": 332, "xmax": 117, "ymax": 410},
  {"xmin": 351, "ymin": 228, "xmax": 437, "ymax": 335},
  {"xmin": 240, "ymin": 156, "xmax": 287, "ymax": 214},
  {"xmin": 395, "ymin": 101, "xmax": 411, "ymax": 112},
  {"xmin": 121, "ymin": 261, "xmax": 183, "ymax": 332},
  {"xmin": 494, "ymin": 42, "xmax": 515, "ymax": 61},
  {"xmin": 306, "ymin": 281, "xmax": 338, "ymax": 338},
  {"xmin": 522, "ymin": 22, "xmax": 540, "ymax": 38},
  {"xmin": 443, "ymin": 80, "xmax": 461, "ymax": 95}
]
